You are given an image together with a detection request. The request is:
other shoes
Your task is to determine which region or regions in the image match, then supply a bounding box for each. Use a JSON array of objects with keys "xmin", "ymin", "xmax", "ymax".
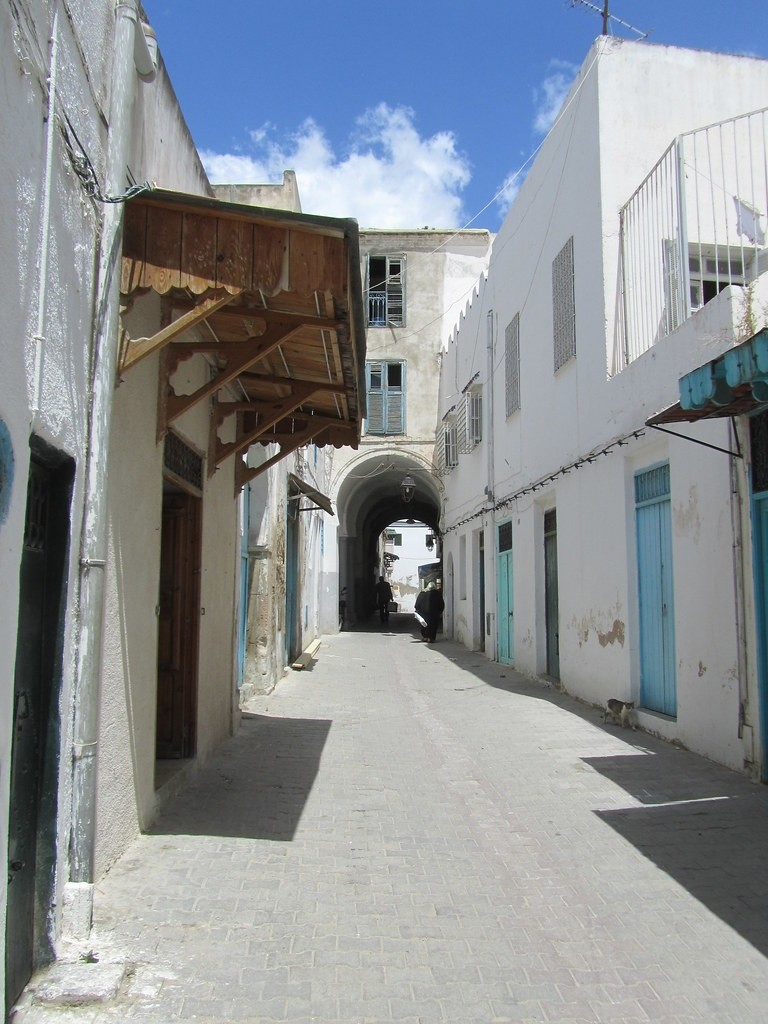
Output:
[
  {"xmin": 382, "ymin": 618, "xmax": 388, "ymax": 622},
  {"xmin": 422, "ymin": 637, "xmax": 433, "ymax": 643}
]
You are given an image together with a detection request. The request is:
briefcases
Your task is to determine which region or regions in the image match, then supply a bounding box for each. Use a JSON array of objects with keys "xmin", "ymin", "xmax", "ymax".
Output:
[{"xmin": 387, "ymin": 600, "xmax": 398, "ymax": 612}]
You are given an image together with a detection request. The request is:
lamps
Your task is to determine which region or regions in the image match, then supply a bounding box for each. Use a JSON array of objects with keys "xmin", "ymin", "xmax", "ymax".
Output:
[{"xmin": 401, "ymin": 476, "xmax": 417, "ymax": 503}]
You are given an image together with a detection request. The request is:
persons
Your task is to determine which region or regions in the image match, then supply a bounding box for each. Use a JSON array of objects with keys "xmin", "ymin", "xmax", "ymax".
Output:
[
  {"xmin": 368, "ymin": 576, "xmax": 394, "ymax": 622},
  {"xmin": 415, "ymin": 582, "xmax": 445, "ymax": 642}
]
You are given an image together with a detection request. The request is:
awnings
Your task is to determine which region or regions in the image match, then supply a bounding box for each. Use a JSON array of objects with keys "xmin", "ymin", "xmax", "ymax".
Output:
[{"xmin": 117, "ymin": 183, "xmax": 369, "ymax": 502}]
